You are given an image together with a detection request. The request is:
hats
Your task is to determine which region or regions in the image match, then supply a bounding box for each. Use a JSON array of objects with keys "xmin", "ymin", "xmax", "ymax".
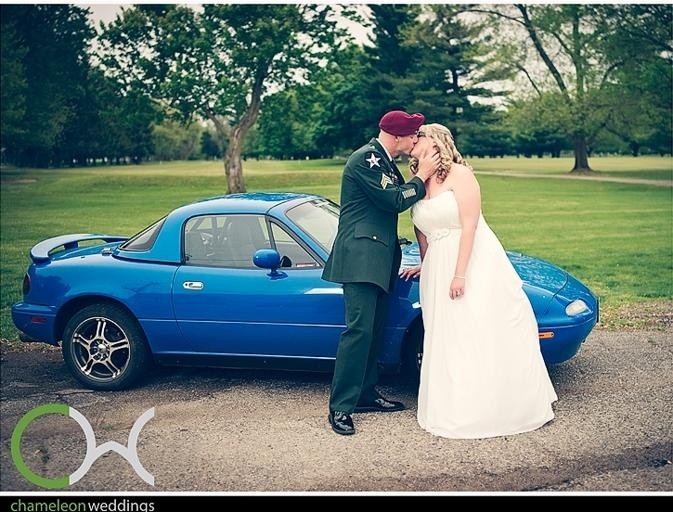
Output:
[{"xmin": 378, "ymin": 111, "xmax": 426, "ymax": 137}]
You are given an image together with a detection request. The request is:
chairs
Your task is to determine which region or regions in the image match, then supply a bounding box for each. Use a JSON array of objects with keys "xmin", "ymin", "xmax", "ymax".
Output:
[
  {"xmin": 184, "ymin": 232, "xmax": 209, "ymax": 260},
  {"xmin": 227, "ymin": 220, "xmax": 257, "ymax": 268}
]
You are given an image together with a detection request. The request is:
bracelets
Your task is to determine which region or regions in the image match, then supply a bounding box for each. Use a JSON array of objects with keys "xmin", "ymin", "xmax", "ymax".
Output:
[{"xmin": 452, "ymin": 275, "xmax": 466, "ymax": 280}]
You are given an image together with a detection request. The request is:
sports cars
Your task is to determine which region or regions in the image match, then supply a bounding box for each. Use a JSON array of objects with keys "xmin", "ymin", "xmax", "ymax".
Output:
[{"xmin": 8, "ymin": 189, "xmax": 607, "ymax": 394}]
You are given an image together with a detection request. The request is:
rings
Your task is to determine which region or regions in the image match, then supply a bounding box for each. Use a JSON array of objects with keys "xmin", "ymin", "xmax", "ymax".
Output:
[{"xmin": 456, "ymin": 291, "xmax": 460, "ymax": 296}]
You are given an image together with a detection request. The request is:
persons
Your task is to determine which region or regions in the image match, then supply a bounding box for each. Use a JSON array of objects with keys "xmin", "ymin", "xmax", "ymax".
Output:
[
  {"xmin": 320, "ymin": 111, "xmax": 441, "ymax": 436},
  {"xmin": 396, "ymin": 122, "xmax": 558, "ymax": 441}
]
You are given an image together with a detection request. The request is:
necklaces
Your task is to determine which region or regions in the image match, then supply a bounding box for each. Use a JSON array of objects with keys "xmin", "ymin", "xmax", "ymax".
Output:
[{"xmin": 428, "ymin": 170, "xmax": 438, "ymax": 179}]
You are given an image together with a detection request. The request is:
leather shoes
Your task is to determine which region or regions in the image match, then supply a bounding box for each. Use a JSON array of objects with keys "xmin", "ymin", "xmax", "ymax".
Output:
[
  {"xmin": 327, "ymin": 406, "xmax": 355, "ymax": 435},
  {"xmin": 353, "ymin": 395, "xmax": 405, "ymax": 413}
]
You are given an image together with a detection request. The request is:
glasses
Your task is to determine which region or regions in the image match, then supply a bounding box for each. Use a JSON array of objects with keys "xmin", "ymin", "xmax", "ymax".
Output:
[{"xmin": 416, "ymin": 130, "xmax": 427, "ymax": 137}]
